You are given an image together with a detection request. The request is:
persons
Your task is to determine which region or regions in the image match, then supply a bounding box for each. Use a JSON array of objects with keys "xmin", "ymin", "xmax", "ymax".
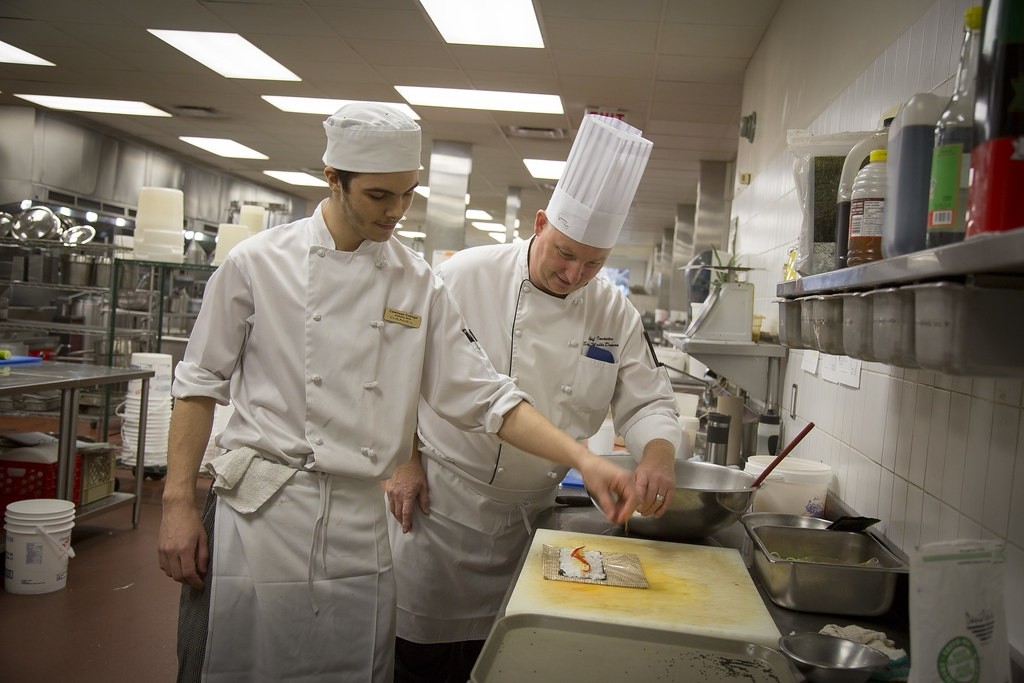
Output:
[
  {"xmin": 382, "ymin": 180, "xmax": 679, "ymax": 683},
  {"xmin": 159, "ymin": 101, "xmax": 634, "ymax": 683}
]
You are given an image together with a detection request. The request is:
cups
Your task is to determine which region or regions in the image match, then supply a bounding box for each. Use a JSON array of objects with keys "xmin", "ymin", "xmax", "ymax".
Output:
[
  {"xmin": 587, "ymin": 422, "xmax": 616, "ymax": 455},
  {"xmin": 705, "ymin": 413, "xmax": 730, "ymax": 466},
  {"xmin": 95, "ymin": 257, "xmax": 112, "ymax": 287},
  {"xmin": 60, "ymin": 253, "xmax": 93, "ymax": 287},
  {"xmin": 752, "ymin": 316, "xmax": 764, "ymax": 343},
  {"xmin": 691, "ymin": 303, "xmax": 704, "ymax": 321},
  {"xmin": 675, "ymin": 416, "xmax": 699, "ymax": 460},
  {"xmin": 654, "ymin": 309, "xmax": 669, "ymax": 324}
]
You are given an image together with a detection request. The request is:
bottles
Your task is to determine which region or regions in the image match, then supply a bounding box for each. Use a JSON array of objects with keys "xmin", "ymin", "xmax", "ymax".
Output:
[
  {"xmin": 963, "ymin": 0, "xmax": 1024, "ymax": 239},
  {"xmin": 847, "ymin": 150, "xmax": 887, "ymax": 267},
  {"xmin": 757, "ymin": 410, "xmax": 780, "ymax": 456},
  {"xmin": 925, "ymin": 5, "xmax": 982, "ymax": 249}
]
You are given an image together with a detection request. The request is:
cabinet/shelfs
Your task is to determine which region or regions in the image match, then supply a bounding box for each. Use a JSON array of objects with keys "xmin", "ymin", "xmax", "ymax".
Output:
[{"xmin": 0, "ymin": 238, "xmax": 219, "ymax": 444}]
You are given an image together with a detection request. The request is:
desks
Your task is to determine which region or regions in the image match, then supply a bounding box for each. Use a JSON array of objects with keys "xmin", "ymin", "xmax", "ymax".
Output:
[
  {"xmin": 0, "ymin": 356, "xmax": 155, "ymax": 530},
  {"xmin": 0, "ymin": 372, "xmax": 77, "ymax": 531}
]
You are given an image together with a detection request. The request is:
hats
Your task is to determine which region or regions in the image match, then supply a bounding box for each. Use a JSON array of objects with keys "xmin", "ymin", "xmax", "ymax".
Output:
[
  {"xmin": 545, "ymin": 114, "xmax": 654, "ymax": 249},
  {"xmin": 321, "ymin": 103, "xmax": 422, "ymax": 173}
]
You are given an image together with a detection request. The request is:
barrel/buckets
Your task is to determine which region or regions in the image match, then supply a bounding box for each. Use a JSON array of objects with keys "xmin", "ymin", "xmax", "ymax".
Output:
[
  {"xmin": 133, "ymin": 187, "xmax": 184, "ymax": 263},
  {"xmin": 4, "ymin": 499, "xmax": 75, "ymax": 595},
  {"xmin": 198, "ymin": 400, "xmax": 236, "ymax": 472},
  {"xmin": 882, "ymin": 94, "xmax": 951, "ymax": 257},
  {"xmin": 211, "ymin": 205, "xmax": 264, "ymax": 266},
  {"xmin": 744, "ymin": 456, "xmax": 831, "ymax": 519},
  {"xmin": 116, "ymin": 353, "xmax": 174, "ymax": 468},
  {"xmin": 834, "ymin": 117, "xmax": 895, "ymax": 269}
]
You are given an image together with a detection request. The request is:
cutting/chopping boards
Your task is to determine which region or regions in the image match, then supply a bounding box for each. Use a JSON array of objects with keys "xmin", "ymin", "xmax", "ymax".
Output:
[{"xmin": 506, "ymin": 529, "xmax": 782, "ymax": 651}]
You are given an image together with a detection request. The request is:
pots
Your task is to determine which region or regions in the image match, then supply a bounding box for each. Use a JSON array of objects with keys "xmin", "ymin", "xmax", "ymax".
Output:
[{"xmin": 49, "ymin": 337, "xmax": 140, "ymax": 393}]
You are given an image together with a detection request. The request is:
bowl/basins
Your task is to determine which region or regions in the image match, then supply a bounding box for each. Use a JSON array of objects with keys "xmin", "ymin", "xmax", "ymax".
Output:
[
  {"xmin": 778, "ymin": 632, "xmax": 889, "ymax": 683},
  {"xmin": 583, "ymin": 453, "xmax": 767, "ymax": 541},
  {"xmin": 117, "ymin": 289, "xmax": 160, "ymax": 311},
  {"xmin": 738, "ymin": 511, "xmax": 835, "ymax": 545}
]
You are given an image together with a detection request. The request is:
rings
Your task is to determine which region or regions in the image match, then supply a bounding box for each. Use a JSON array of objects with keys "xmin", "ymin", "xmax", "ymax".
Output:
[{"xmin": 656, "ymin": 494, "xmax": 665, "ymax": 502}]
